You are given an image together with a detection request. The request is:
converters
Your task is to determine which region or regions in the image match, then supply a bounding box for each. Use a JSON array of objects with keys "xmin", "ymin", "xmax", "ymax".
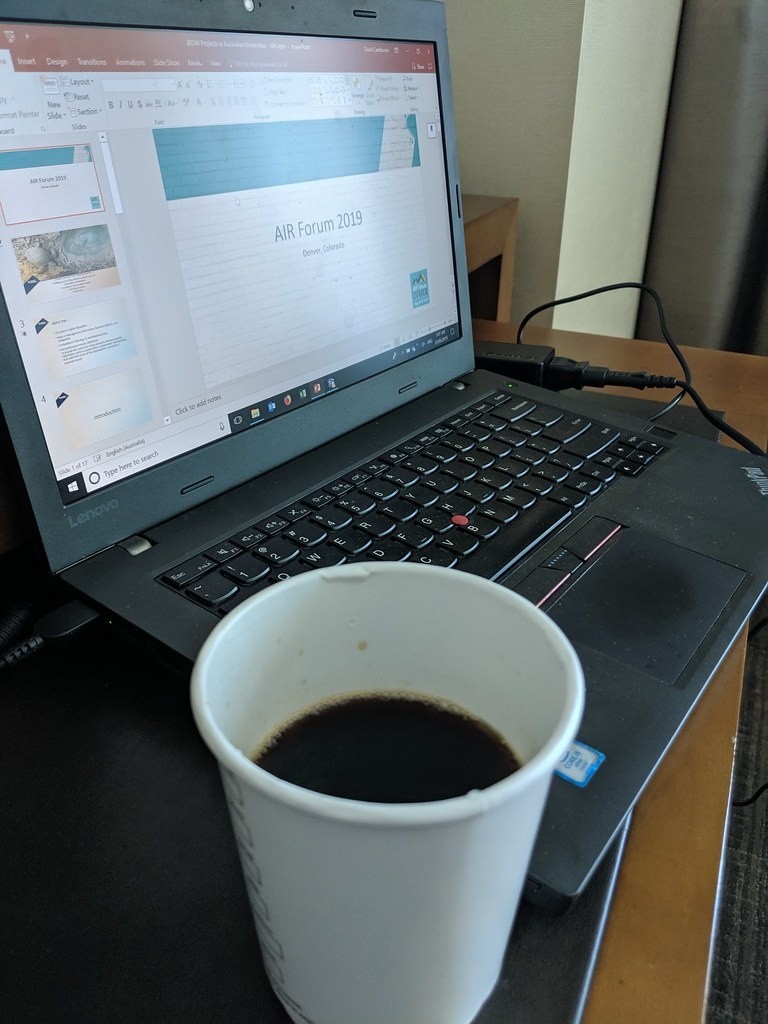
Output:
[{"xmin": 473, "ymin": 339, "xmax": 555, "ymax": 389}]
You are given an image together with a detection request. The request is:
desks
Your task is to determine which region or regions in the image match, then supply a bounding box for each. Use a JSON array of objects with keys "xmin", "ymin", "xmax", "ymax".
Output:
[
  {"xmin": 472, "ymin": 317, "xmax": 768, "ymax": 1024},
  {"xmin": 462, "ymin": 194, "xmax": 519, "ymax": 322}
]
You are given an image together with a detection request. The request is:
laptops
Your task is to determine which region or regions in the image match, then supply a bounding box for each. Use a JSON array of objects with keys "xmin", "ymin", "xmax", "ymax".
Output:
[{"xmin": 0, "ymin": 0, "xmax": 767, "ymax": 918}]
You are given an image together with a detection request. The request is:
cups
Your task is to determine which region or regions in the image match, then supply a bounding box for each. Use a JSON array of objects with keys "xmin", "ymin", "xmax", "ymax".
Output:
[{"xmin": 190, "ymin": 562, "xmax": 583, "ymax": 1024}]
